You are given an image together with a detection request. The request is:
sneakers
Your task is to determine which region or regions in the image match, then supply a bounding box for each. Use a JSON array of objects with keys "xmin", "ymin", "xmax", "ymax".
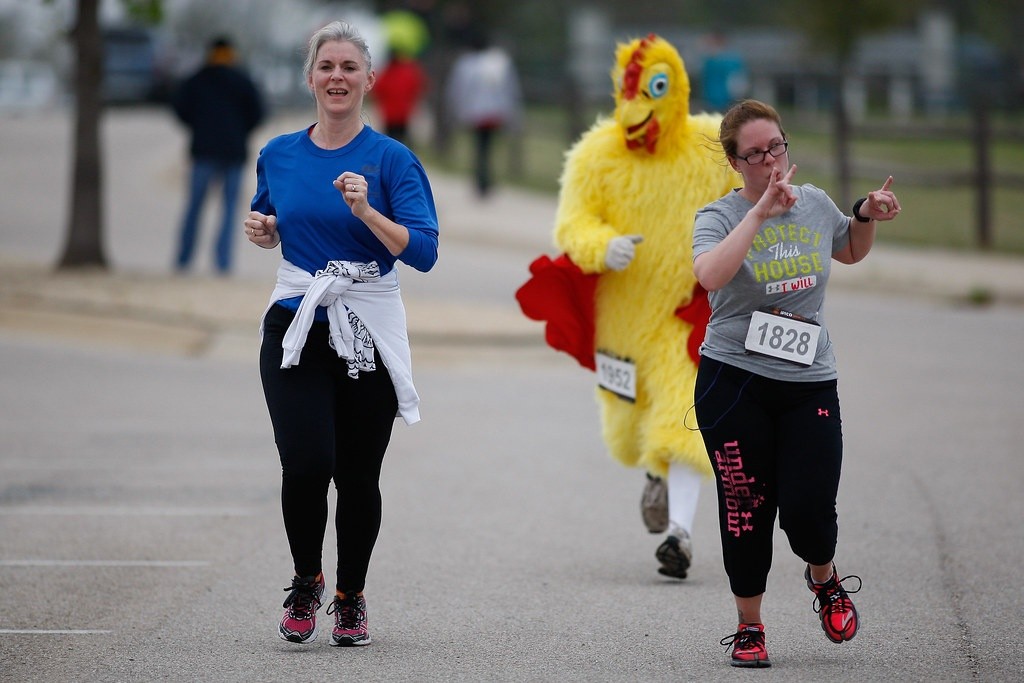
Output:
[
  {"xmin": 642, "ymin": 481, "xmax": 668, "ymax": 533},
  {"xmin": 329, "ymin": 594, "xmax": 371, "ymax": 646},
  {"xmin": 730, "ymin": 623, "xmax": 772, "ymax": 667},
  {"xmin": 804, "ymin": 561, "xmax": 860, "ymax": 644},
  {"xmin": 655, "ymin": 529, "xmax": 691, "ymax": 579},
  {"xmin": 278, "ymin": 571, "xmax": 326, "ymax": 643}
]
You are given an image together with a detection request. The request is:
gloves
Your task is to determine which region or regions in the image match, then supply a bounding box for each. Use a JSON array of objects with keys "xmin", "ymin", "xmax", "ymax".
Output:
[{"xmin": 605, "ymin": 235, "xmax": 644, "ymax": 272}]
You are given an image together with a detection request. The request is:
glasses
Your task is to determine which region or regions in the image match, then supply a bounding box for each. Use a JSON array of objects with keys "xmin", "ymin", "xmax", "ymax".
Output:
[{"xmin": 731, "ymin": 139, "xmax": 788, "ymax": 165}]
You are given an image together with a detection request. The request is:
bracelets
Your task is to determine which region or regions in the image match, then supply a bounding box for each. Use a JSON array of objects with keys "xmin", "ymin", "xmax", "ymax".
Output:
[{"xmin": 852, "ymin": 197, "xmax": 876, "ymax": 224}]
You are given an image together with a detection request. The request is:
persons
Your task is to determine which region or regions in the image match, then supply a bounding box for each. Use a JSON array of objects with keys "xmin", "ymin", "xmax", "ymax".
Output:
[
  {"xmin": 689, "ymin": 97, "xmax": 905, "ymax": 677},
  {"xmin": 371, "ymin": 35, "xmax": 425, "ymax": 151},
  {"xmin": 449, "ymin": 29, "xmax": 523, "ymax": 197},
  {"xmin": 242, "ymin": 19, "xmax": 443, "ymax": 652},
  {"xmin": 167, "ymin": 32, "xmax": 269, "ymax": 273}
]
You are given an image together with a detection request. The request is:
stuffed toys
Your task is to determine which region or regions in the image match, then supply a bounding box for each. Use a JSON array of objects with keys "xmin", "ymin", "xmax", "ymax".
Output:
[{"xmin": 550, "ymin": 30, "xmax": 752, "ymax": 580}]
